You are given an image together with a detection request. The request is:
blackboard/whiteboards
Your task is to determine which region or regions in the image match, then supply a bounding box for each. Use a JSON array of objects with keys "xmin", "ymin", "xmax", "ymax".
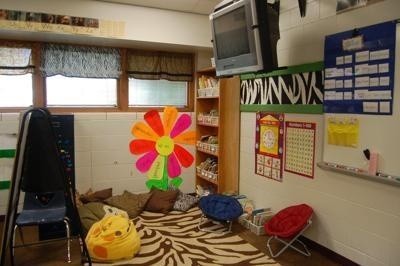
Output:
[{"xmin": 317, "ymin": 19, "xmax": 400, "ymax": 186}]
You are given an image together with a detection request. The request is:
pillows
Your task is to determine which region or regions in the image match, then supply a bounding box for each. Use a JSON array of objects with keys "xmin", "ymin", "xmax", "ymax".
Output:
[{"xmin": 76, "ymin": 187, "xmax": 199, "ymax": 264}]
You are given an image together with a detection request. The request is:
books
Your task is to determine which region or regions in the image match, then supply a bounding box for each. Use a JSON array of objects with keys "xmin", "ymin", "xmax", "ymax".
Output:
[
  {"xmin": 194, "ymin": 74, "xmax": 219, "ymax": 198},
  {"xmin": 242, "ymin": 207, "xmax": 276, "ymax": 236}
]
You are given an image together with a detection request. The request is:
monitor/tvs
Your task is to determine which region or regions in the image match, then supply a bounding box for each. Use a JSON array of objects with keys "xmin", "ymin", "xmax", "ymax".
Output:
[{"xmin": 209, "ymin": 0, "xmax": 279, "ymax": 76}]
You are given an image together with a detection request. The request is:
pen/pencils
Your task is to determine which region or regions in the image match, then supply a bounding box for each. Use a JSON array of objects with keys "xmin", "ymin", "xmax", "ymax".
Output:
[{"xmin": 325, "ymin": 163, "xmax": 400, "ymax": 181}]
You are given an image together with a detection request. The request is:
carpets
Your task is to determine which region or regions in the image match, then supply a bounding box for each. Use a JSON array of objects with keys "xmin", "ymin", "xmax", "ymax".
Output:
[{"xmin": 78, "ymin": 207, "xmax": 281, "ymax": 266}]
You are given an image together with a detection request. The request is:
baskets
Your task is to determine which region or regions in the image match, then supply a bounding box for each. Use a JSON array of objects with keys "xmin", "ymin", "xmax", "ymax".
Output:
[{"xmin": 240, "ymin": 216, "xmax": 267, "ymax": 236}]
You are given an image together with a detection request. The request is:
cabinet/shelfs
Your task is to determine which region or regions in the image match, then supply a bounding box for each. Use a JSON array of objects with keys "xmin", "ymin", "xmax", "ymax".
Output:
[{"xmin": 193, "ymin": 67, "xmax": 241, "ymax": 200}]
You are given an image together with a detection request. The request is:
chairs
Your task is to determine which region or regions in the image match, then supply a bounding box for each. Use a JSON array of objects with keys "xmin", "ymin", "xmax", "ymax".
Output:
[
  {"xmin": 197, "ymin": 193, "xmax": 243, "ymax": 235},
  {"xmin": 13, "ymin": 189, "xmax": 71, "ymax": 264},
  {"xmin": 264, "ymin": 204, "xmax": 314, "ymax": 258}
]
